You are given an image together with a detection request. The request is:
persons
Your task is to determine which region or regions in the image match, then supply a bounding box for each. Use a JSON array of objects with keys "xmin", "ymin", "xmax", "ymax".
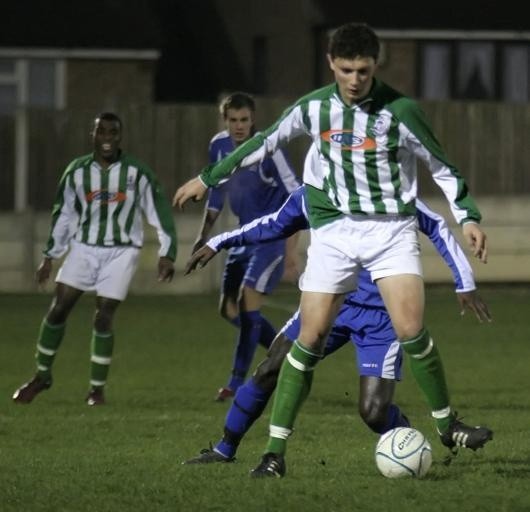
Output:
[
  {"xmin": 172, "ymin": 21, "xmax": 494, "ymax": 479},
  {"xmin": 12, "ymin": 113, "xmax": 177, "ymax": 407},
  {"xmin": 182, "ymin": 185, "xmax": 494, "ymax": 466},
  {"xmin": 192, "ymin": 89, "xmax": 301, "ymax": 404}
]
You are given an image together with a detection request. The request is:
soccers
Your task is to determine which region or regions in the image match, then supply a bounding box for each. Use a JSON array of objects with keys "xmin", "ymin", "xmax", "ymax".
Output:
[{"xmin": 375, "ymin": 428, "xmax": 432, "ymax": 479}]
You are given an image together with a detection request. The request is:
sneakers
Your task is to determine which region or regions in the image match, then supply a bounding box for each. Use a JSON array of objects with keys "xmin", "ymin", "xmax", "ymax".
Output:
[
  {"xmin": 250, "ymin": 454, "xmax": 285, "ymax": 479},
  {"xmin": 182, "ymin": 448, "xmax": 237, "ymax": 464},
  {"xmin": 12, "ymin": 376, "xmax": 53, "ymax": 404},
  {"xmin": 86, "ymin": 392, "xmax": 105, "ymax": 407},
  {"xmin": 440, "ymin": 417, "xmax": 494, "ymax": 451},
  {"xmin": 213, "ymin": 385, "xmax": 238, "ymax": 401}
]
